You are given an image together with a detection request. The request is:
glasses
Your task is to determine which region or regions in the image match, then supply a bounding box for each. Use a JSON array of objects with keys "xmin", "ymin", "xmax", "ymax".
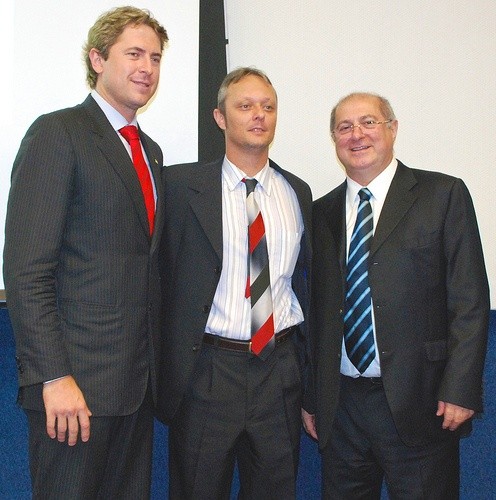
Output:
[{"xmin": 332, "ymin": 116, "xmax": 391, "ymax": 135}]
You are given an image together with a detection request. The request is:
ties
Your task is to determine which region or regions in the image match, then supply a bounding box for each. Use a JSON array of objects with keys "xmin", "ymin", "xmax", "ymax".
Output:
[
  {"xmin": 241, "ymin": 178, "xmax": 287, "ymax": 371},
  {"xmin": 343, "ymin": 188, "xmax": 376, "ymax": 374},
  {"xmin": 119, "ymin": 125, "xmax": 155, "ymax": 239}
]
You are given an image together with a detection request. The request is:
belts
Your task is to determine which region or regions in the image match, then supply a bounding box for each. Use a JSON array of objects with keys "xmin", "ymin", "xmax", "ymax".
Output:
[
  {"xmin": 203, "ymin": 327, "xmax": 294, "ymax": 355},
  {"xmin": 340, "ymin": 374, "xmax": 383, "ymax": 386}
]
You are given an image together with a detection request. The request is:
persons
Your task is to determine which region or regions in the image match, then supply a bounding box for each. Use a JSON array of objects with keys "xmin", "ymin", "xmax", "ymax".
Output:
[
  {"xmin": 2, "ymin": 3, "xmax": 170, "ymax": 500},
  {"xmin": 150, "ymin": 66, "xmax": 316, "ymax": 500},
  {"xmin": 296, "ymin": 91, "xmax": 493, "ymax": 500}
]
